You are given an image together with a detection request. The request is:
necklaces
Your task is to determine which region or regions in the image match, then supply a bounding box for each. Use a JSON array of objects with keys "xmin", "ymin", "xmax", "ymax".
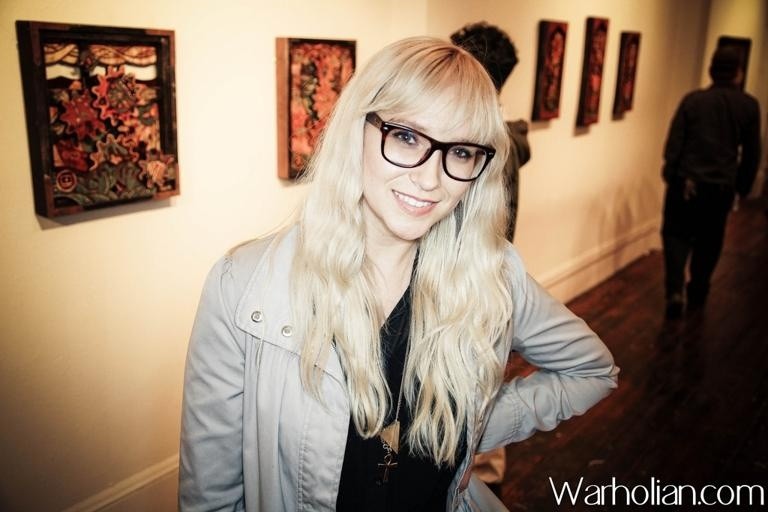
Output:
[{"xmin": 379, "ymin": 270, "xmax": 412, "ymax": 487}]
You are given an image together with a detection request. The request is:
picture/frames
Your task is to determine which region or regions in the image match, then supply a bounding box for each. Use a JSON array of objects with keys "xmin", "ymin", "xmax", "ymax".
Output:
[
  {"xmin": 613, "ymin": 32, "xmax": 641, "ymax": 116},
  {"xmin": 531, "ymin": 19, "xmax": 569, "ymax": 121},
  {"xmin": 14, "ymin": 19, "xmax": 180, "ymax": 218},
  {"xmin": 275, "ymin": 37, "xmax": 357, "ymax": 182},
  {"xmin": 576, "ymin": 16, "xmax": 609, "ymax": 127}
]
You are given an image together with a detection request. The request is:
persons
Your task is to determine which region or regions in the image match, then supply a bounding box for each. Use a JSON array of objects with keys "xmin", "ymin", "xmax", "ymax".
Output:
[
  {"xmin": 176, "ymin": 36, "xmax": 622, "ymax": 512},
  {"xmin": 446, "ymin": 21, "xmax": 532, "ymax": 366},
  {"xmin": 657, "ymin": 46, "xmax": 764, "ymax": 328}
]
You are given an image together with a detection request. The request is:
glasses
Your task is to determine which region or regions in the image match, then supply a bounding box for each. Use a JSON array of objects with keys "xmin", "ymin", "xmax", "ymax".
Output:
[{"xmin": 366, "ymin": 112, "xmax": 497, "ymax": 184}]
